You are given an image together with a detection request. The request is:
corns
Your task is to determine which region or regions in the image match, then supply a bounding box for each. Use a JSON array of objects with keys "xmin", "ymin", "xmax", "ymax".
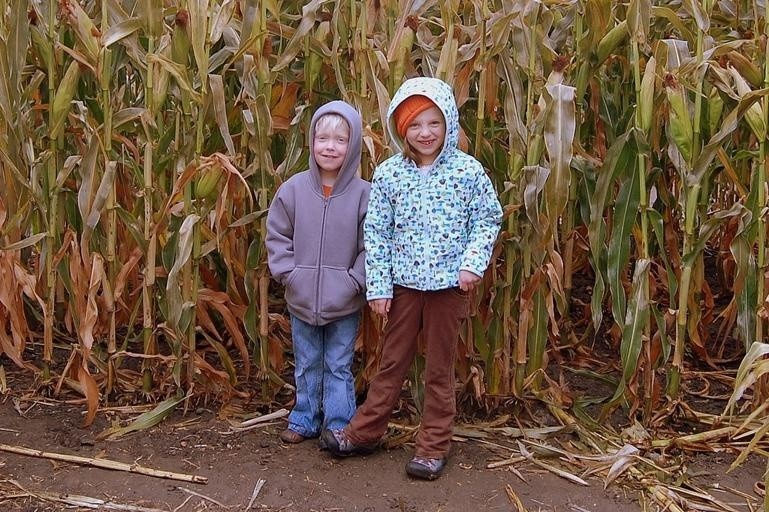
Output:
[{"xmin": 29, "ymin": 0, "xmax": 765, "ymax": 196}]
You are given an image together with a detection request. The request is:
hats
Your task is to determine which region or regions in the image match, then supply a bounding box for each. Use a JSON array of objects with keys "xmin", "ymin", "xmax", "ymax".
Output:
[{"xmin": 394, "ymin": 94, "xmax": 437, "ymax": 141}]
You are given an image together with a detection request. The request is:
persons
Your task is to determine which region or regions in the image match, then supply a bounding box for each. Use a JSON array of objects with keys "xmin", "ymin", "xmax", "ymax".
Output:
[
  {"xmin": 319, "ymin": 74, "xmax": 506, "ymax": 482},
  {"xmin": 264, "ymin": 98, "xmax": 375, "ymax": 454}
]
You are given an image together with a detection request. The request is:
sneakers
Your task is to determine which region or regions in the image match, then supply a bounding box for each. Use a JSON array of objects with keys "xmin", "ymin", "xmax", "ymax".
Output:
[
  {"xmin": 281, "ymin": 428, "xmax": 307, "ymax": 443},
  {"xmin": 405, "ymin": 450, "xmax": 447, "ymax": 482},
  {"xmin": 320, "ymin": 425, "xmax": 367, "ymax": 457}
]
